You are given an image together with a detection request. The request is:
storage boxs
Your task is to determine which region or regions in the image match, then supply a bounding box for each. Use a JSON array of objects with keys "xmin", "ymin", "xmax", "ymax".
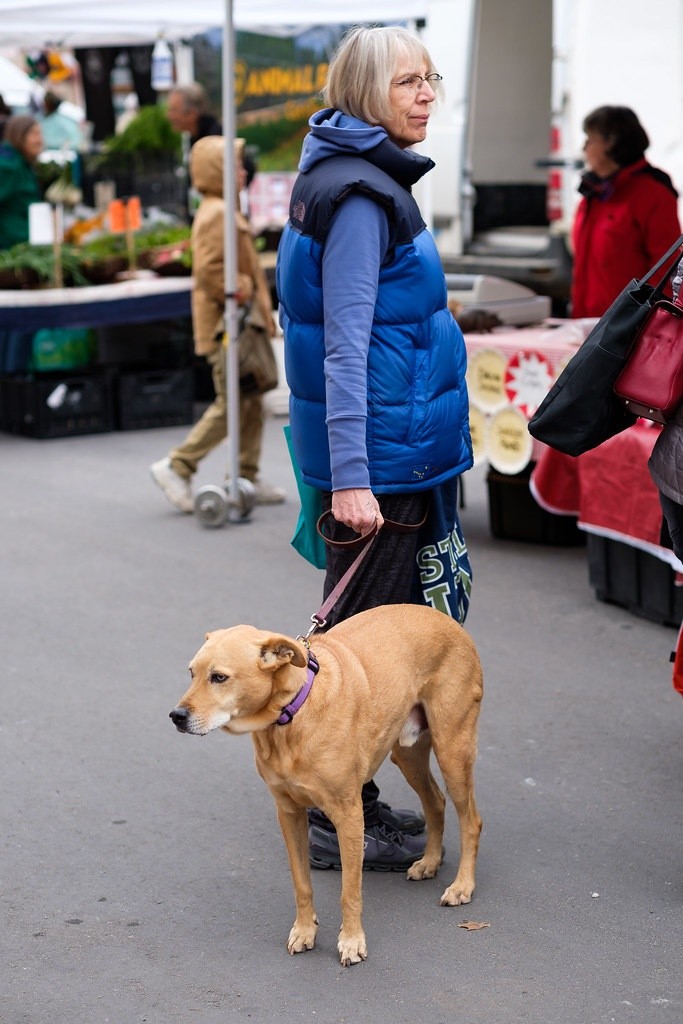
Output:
[
  {"xmin": 0, "ymin": 364, "xmax": 114, "ymax": 438},
  {"xmin": 487, "ymin": 461, "xmax": 578, "ymax": 546},
  {"xmin": 116, "ymin": 368, "xmax": 193, "ymax": 430},
  {"xmin": 587, "ymin": 532, "xmax": 683, "ymax": 630}
]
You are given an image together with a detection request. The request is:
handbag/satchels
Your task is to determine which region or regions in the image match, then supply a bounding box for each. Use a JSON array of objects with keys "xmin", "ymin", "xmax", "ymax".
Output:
[
  {"xmin": 528, "ymin": 233, "xmax": 683, "ymax": 457},
  {"xmin": 283, "ymin": 424, "xmax": 327, "ymax": 568},
  {"xmin": 219, "ymin": 329, "xmax": 280, "ymax": 396}
]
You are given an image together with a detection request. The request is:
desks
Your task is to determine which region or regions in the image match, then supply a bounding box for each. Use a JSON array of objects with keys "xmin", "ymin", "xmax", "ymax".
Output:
[
  {"xmin": 0, "ymin": 277, "xmax": 192, "ymax": 371},
  {"xmin": 461, "ymin": 317, "xmax": 605, "ymax": 479}
]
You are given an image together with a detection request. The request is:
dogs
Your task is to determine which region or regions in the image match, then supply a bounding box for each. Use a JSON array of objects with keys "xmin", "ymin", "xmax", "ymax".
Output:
[{"xmin": 168, "ymin": 603, "xmax": 484, "ymax": 967}]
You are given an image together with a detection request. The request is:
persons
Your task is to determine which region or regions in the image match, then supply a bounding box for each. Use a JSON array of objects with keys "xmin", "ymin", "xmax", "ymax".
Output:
[
  {"xmin": 570, "ymin": 106, "xmax": 683, "ymax": 318},
  {"xmin": 150, "ymin": 135, "xmax": 288, "ymax": 513},
  {"xmin": 0, "ymin": 115, "xmax": 44, "ymax": 250},
  {"xmin": 648, "ymin": 251, "xmax": 683, "ymax": 695},
  {"xmin": 274, "ymin": 25, "xmax": 476, "ymax": 873},
  {"xmin": 87, "ymin": 81, "xmax": 257, "ymax": 226}
]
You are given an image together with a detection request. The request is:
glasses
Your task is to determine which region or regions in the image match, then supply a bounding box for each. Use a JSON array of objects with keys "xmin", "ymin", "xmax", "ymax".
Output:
[{"xmin": 390, "ymin": 73, "xmax": 444, "ymax": 92}]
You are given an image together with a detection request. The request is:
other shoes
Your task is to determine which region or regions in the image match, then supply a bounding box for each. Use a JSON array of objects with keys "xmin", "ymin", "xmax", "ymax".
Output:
[
  {"xmin": 253, "ymin": 482, "xmax": 285, "ymax": 505},
  {"xmin": 150, "ymin": 457, "xmax": 194, "ymax": 513}
]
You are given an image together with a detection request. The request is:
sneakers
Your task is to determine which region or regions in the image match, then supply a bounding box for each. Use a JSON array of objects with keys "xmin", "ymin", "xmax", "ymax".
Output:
[
  {"xmin": 307, "ymin": 801, "xmax": 425, "ymax": 836},
  {"xmin": 307, "ymin": 824, "xmax": 445, "ymax": 872}
]
